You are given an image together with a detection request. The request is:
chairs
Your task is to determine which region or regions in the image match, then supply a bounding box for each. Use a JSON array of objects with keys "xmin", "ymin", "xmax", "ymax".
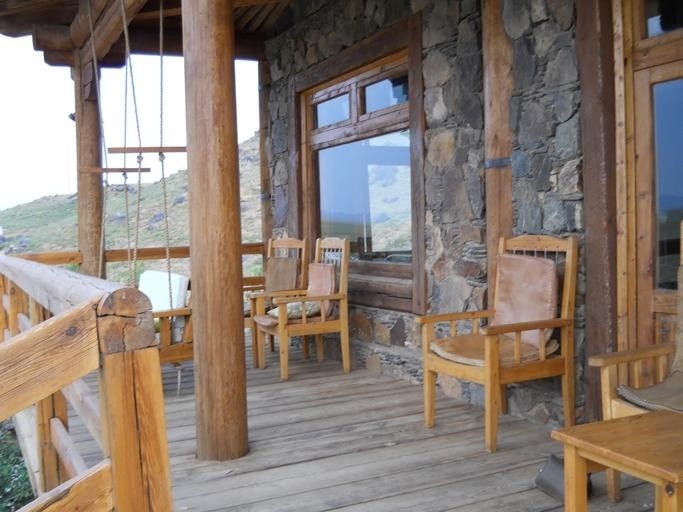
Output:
[
  {"xmin": 243, "ymin": 236, "xmax": 309, "ymax": 366},
  {"xmin": 588, "ymin": 220, "xmax": 683, "ymax": 505},
  {"xmin": 414, "ymin": 234, "xmax": 578, "ymax": 454},
  {"xmin": 253, "ymin": 237, "xmax": 351, "ymax": 381}
]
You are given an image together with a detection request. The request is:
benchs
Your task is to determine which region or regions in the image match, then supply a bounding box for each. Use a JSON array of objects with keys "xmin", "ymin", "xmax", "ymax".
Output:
[{"xmin": 138, "ymin": 270, "xmax": 193, "ymax": 365}]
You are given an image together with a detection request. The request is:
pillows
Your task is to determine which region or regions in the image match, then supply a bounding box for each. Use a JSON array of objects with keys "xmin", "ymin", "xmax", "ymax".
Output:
[{"xmin": 265, "ymin": 301, "xmax": 320, "ymax": 320}]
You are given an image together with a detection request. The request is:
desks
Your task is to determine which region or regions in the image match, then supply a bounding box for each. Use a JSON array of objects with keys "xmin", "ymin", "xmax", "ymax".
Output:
[{"xmin": 551, "ymin": 410, "xmax": 683, "ymax": 512}]
